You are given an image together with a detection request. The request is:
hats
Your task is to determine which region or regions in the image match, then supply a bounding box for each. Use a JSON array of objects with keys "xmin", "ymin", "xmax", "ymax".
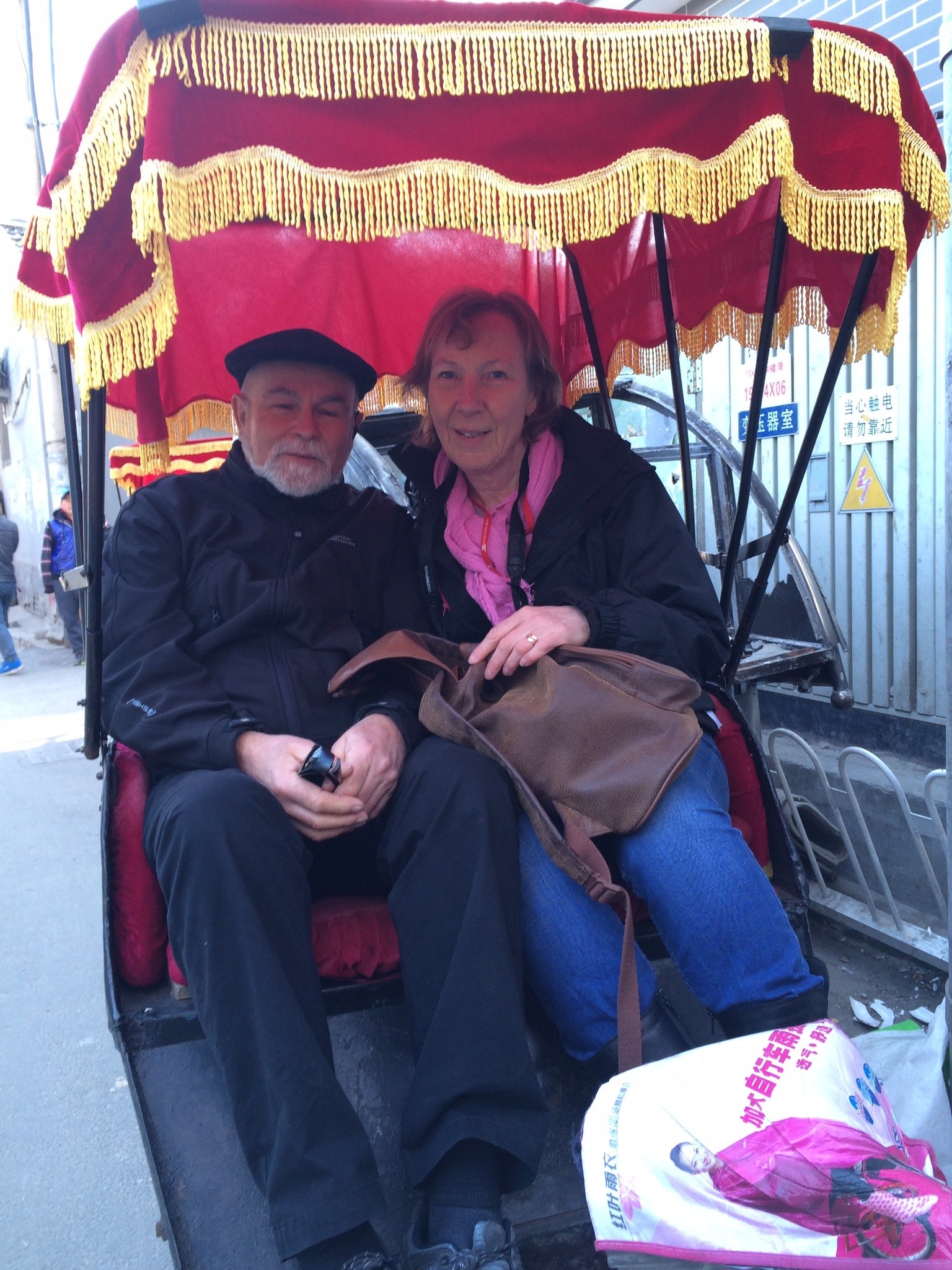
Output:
[
  {"xmin": 60, "ymin": 487, "xmax": 70, "ymax": 500},
  {"xmin": 224, "ymin": 328, "xmax": 378, "ymax": 401}
]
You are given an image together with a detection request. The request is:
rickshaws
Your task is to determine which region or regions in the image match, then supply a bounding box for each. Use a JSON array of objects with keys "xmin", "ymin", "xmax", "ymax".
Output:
[{"xmin": 2, "ymin": 1, "xmax": 949, "ymax": 1270}]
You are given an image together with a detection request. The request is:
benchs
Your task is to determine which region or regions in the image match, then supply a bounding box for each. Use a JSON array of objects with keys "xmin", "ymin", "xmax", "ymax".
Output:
[{"xmin": 103, "ymin": 523, "xmax": 778, "ymax": 1049}]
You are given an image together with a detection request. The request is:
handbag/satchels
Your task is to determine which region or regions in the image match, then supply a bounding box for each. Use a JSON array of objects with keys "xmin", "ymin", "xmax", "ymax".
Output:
[{"xmin": 580, "ymin": 1018, "xmax": 952, "ymax": 1270}]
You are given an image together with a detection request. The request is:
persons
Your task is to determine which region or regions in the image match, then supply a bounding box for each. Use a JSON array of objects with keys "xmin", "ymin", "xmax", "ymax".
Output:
[
  {"xmin": 99, "ymin": 328, "xmax": 616, "ymax": 1270},
  {"xmin": 670, "ymin": 1117, "xmax": 939, "ymax": 1235},
  {"xmin": 391, "ymin": 285, "xmax": 832, "ymax": 1116},
  {"xmin": 0, "ymin": 502, "xmax": 24, "ymax": 677},
  {"xmin": 40, "ymin": 487, "xmax": 86, "ymax": 667}
]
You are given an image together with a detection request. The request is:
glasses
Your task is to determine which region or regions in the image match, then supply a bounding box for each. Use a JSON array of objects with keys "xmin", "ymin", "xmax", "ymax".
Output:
[{"xmin": 298, "ymin": 743, "xmax": 342, "ymax": 794}]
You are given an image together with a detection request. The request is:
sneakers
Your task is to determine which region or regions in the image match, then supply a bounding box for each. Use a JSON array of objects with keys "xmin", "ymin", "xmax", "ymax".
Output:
[{"xmin": 0, "ymin": 658, "xmax": 24, "ymax": 676}]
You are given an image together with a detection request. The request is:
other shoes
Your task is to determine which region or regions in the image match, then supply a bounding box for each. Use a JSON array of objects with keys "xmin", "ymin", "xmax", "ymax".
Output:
[
  {"xmin": 396, "ymin": 1195, "xmax": 524, "ymax": 1270},
  {"xmin": 280, "ymin": 1253, "xmax": 396, "ymax": 1270},
  {"xmin": 75, "ymin": 652, "xmax": 86, "ymax": 665}
]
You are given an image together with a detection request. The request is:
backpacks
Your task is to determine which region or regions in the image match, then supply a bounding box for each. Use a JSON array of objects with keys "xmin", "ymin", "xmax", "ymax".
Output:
[{"xmin": 327, "ymin": 629, "xmax": 703, "ymax": 905}]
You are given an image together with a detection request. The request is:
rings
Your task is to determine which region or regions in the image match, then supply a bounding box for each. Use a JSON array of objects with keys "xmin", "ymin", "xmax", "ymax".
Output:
[{"xmin": 525, "ymin": 634, "xmax": 538, "ymax": 645}]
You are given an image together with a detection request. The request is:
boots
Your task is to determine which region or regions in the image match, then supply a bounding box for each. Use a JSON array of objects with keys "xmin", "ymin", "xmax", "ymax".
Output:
[
  {"xmin": 706, "ymin": 955, "xmax": 830, "ymax": 1041},
  {"xmin": 585, "ymin": 987, "xmax": 698, "ymax": 1087}
]
